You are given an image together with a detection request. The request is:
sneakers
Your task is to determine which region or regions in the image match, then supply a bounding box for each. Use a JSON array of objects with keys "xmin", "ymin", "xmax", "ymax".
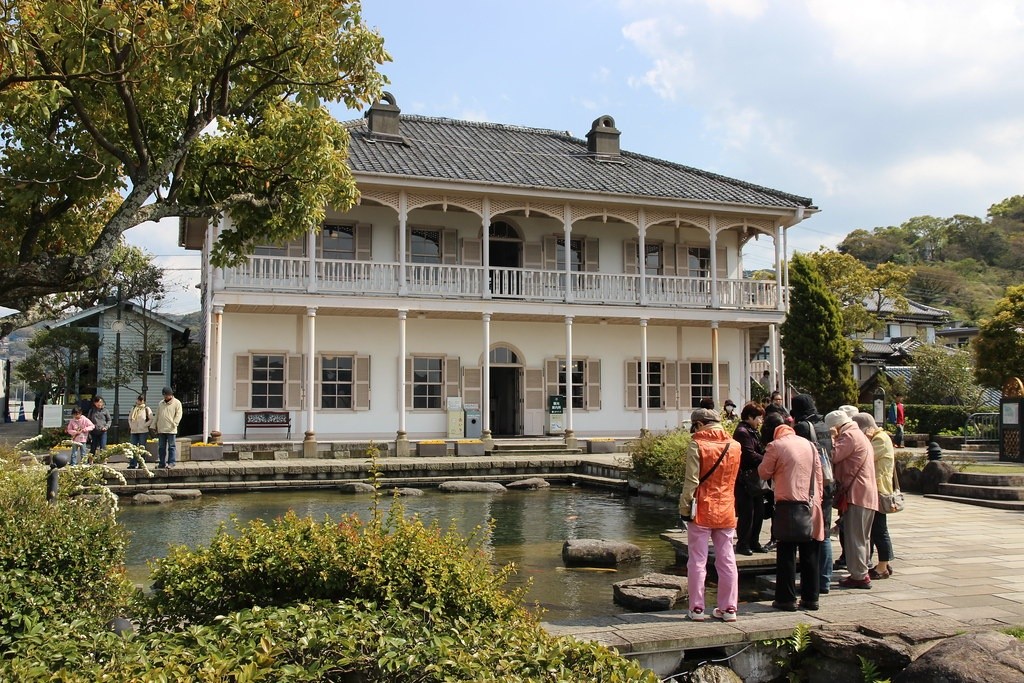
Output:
[
  {"xmin": 713, "ymin": 608, "xmax": 736, "ymax": 621},
  {"xmin": 688, "ymin": 608, "xmax": 706, "ymax": 621}
]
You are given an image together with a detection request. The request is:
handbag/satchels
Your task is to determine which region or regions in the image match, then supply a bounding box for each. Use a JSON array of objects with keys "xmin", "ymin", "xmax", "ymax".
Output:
[
  {"xmin": 148, "ymin": 428, "xmax": 157, "ymax": 439},
  {"xmin": 681, "ymin": 497, "xmax": 695, "ymax": 522},
  {"xmin": 807, "ymin": 420, "xmax": 834, "ymax": 487},
  {"xmin": 837, "ymin": 492, "xmax": 848, "ymax": 517},
  {"xmin": 813, "ymin": 504, "xmax": 824, "ymax": 541},
  {"xmin": 878, "ymin": 489, "xmax": 905, "ymax": 514},
  {"xmin": 762, "ymin": 480, "xmax": 773, "ymax": 519}
]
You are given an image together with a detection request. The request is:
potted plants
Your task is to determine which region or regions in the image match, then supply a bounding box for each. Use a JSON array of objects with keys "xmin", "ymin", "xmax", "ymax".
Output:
[
  {"xmin": 587, "ymin": 438, "xmax": 615, "ymax": 454},
  {"xmin": 190, "ymin": 442, "xmax": 223, "ymax": 461},
  {"xmin": 417, "ymin": 440, "xmax": 448, "ymax": 457},
  {"xmin": 454, "ymin": 440, "xmax": 484, "ymax": 457},
  {"xmin": 145, "ymin": 439, "xmax": 181, "ymax": 462}
]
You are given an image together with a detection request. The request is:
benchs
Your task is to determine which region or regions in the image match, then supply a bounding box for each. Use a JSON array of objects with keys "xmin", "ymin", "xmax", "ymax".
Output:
[{"xmin": 244, "ymin": 412, "xmax": 292, "ymax": 440}]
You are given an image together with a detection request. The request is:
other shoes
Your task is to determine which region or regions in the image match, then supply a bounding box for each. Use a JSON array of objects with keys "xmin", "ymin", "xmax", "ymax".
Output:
[
  {"xmin": 899, "ymin": 445, "xmax": 905, "ymax": 448},
  {"xmin": 127, "ymin": 465, "xmax": 135, "ymax": 469},
  {"xmin": 737, "ymin": 540, "xmax": 893, "ymax": 611},
  {"xmin": 894, "ymin": 443, "xmax": 898, "ymax": 448},
  {"xmin": 154, "ymin": 465, "xmax": 166, "ymax": 470},
  {"xmin": 167, "ymin": 463, "xmax": 176, "ymax": 469}
]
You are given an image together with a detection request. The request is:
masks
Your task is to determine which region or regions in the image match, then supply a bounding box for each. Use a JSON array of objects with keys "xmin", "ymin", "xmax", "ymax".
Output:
[{"xmin": 726, "ymin": 406, "xmax": 733, "ymax": 411}]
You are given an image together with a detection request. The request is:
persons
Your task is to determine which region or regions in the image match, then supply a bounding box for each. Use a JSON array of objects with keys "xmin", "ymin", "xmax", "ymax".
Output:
[
  {"xmin": 679, "ymin": 408, "xmax": 742, "ymax": 622},
  {"xmin": 733, "ymin": 391, "xmax": 833, "ymax": 611},
  {"xmin": 127, "ymin": 395, "xmax": 153, "ymax": 469},
  {"xmin": 32, "ymin": 372, "xmax": 51, "ymax": 421},
  {"xmin": 833, "ymin": 405, "xmax": 874, "ymax": 570},
  {"xmin": 87, "ymin": 396, "xmax": 112, "ymax": 465},
  {"xmin": 149, "ymin": 387, "xmax": 182, "ymax": 469},
  {"xmin": 889, "ymin": 393, "xmax": 906, "ymax": 448},
  {"xmin": 720, "ymin": 399, "xmax": 739, "ymax": 421},
  {"xmin": 852, "ymin": 412, "xmax": 895, "ymax": 580},
  {"xmin": 760, "ymin": 370, "xmax": 770, "ymax": 387},
  {"xmin": 690, "ymin": 398, "xmax": 715, "ymax": 433},
  {"xmin": 824, "ymin": 410, "xmax": 879, "ymax": 589},
  {"xmin": 67, "ymin": 406, "xmax": 95, "ymax": 466}
]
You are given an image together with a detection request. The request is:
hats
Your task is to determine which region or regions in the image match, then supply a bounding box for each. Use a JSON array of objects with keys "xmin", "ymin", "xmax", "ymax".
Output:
[
  {"xmin": 840, "ymin": 405, "xmax": 859, "ymax": 419},
  {"xmin": 826, "ymin": 410, "xmax": 853, "ymax": 429},
  {"xmin": 790, "ymin": 396, "xmax": 817, "ymax": 416},
  {"xmin": 690, "ymin": 399, "xmax": 721, "ymax": 433},
  {"xmin": 724, "ymin": 399, "xmax": 736, "ymax": 411},
  {"xmin": 162, "ymin": 386, "xmax": 174, "ymax": 395},
  {"xmin": 853, "ymin": 413, "xmax": 878, "ymax": 434}
]
